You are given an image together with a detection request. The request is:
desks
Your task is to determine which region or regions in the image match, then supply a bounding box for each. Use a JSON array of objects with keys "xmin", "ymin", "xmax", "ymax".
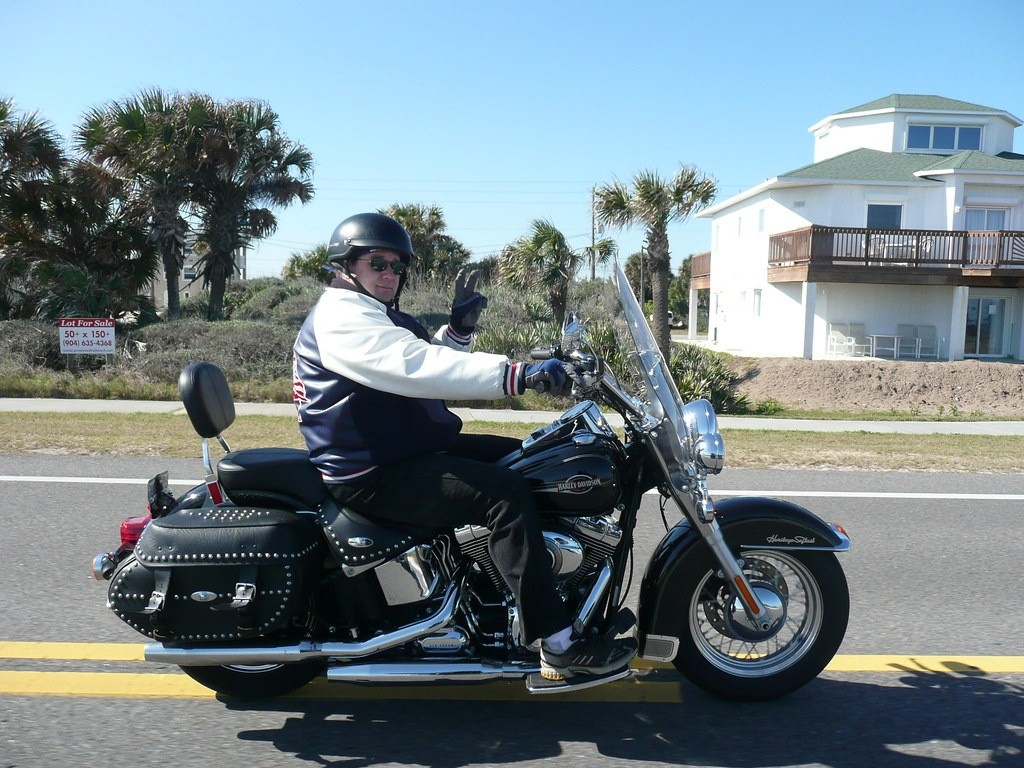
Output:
[
  {"xmin": 881, "ymin": 244, "xmax": 914, "ymax": 258},
  {"xmin": 870, "ymin": 334, "xmax": 903, "ymax": 359}
]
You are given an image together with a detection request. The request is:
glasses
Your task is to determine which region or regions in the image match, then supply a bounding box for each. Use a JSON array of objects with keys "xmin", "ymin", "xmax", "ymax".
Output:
[{"xmin": 355, "ymin": 257, "xmax": 405, "ymax": 276}]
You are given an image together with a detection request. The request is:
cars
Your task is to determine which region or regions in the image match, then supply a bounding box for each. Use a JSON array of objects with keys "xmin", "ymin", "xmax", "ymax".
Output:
[{"xmin": 648, "ymin": 309, "xmax": 674, "ymax": 327}]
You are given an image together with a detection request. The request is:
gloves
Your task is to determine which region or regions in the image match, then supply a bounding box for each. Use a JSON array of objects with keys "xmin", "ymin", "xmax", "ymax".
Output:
[
  {"xmin": 450, "ymin": 267, "xmax": 488, "ymax": 336},
  {"xmin": 525, "ymin": 358, "xmax": 573, "ymax": 398}
]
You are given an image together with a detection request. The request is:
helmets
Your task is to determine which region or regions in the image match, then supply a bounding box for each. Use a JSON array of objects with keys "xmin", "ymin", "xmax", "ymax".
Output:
[{"xmin": 327, "ymin": 213, "xmax": 414, "ymax": 268}]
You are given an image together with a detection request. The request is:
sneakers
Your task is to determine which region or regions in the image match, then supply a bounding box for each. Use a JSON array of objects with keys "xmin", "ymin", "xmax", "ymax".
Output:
[{"xmin": 540, "ymin": 632, "xmax": 638, "ymax": 680}]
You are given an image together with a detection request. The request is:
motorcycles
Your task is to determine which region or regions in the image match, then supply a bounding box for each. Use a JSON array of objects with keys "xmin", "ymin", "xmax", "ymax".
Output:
[{"xmin": 90, "ymin": 262, "xmax": 854, "ymax": 706}]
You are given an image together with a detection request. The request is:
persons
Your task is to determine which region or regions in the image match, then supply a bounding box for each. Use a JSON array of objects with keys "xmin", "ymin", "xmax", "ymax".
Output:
[{"xmin": 292, "ymin": 213, "xmax": 638, "ymax": 682}]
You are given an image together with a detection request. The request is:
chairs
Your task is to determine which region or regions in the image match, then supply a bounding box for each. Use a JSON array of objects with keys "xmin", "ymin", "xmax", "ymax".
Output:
[
  {"xmin": 828, "ymin": 323, "xmax": 872, "ymax": 357},
  {"xmin": 903, "ymin": 238, "xmax": 934, "ymax": 259},
  {"xmin": 862, "ymin": 237, "xmax": 885, "ymax": 258},
  {"xmin": 917, "ymin": 326, "xmax": 945, "ymax": 359},
  {"xmin": 897, "ymin": 324, "xmax": 918, "ymax": 359}
]
